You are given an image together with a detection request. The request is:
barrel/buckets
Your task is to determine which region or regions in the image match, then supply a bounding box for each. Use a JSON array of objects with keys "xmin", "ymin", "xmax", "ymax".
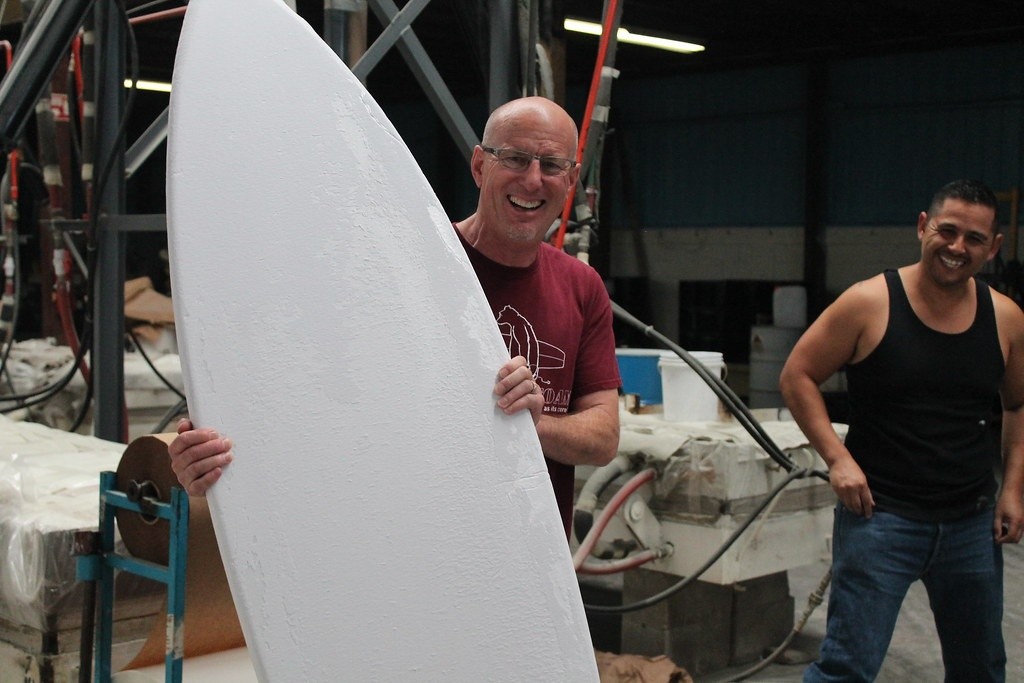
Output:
[
  {"xmin": 658, "ymin": 351, "xmax": 724, "ymax": 422},
  {"xmin": 749, "ymin": 325, "xmax": 804, "ymax": 409}
]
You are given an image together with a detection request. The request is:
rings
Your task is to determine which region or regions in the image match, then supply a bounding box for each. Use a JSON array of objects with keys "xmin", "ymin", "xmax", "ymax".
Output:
[{"xmin": 530, "ymin": 380, "xmax": 536, "ymax": 393}]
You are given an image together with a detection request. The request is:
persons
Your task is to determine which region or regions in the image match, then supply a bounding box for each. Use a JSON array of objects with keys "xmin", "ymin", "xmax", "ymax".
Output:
[
  {"xmin": 167, "ymin": 94, "xmax": 622, "ymax": 549},
  {"xmin": 782, "ymin": 182, "xmax": 1024, "ymax": 683}
]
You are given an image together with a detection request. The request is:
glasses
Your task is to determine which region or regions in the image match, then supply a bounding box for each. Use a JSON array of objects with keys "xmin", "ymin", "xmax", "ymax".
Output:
[{"xmin": 483, "ymin": 147, "xmax": 576, "ymax": 176}]
